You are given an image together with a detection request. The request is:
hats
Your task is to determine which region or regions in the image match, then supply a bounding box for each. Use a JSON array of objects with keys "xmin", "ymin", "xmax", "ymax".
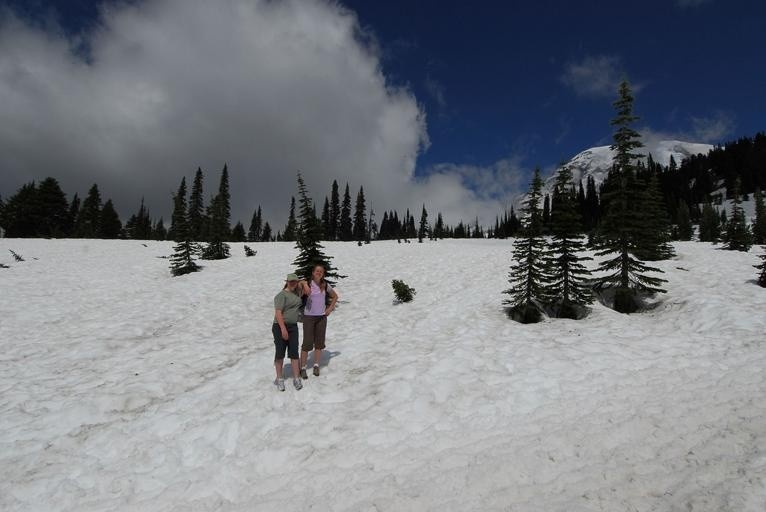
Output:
[{"xmin": 287, "ymin": 273, "xmax": 298, "ymax": 282}]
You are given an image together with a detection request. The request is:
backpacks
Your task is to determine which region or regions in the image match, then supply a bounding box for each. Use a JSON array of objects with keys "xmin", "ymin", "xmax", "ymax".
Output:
[{"xmin": 302, "ymin": 278, "xmax": 329, "ymax": 306}]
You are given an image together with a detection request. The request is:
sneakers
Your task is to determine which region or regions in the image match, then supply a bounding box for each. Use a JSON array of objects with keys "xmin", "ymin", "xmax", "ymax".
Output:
[
  {"xmin": 293, "ymin": 379, "xmax": 302, "ymax": 390},
  {"xmin": 300, "ymin": 366, "xmax": 319, "ymax": 379},
  {"xmin": 274, "ymin": 378, "xmax": 285, "ymax": 391}
]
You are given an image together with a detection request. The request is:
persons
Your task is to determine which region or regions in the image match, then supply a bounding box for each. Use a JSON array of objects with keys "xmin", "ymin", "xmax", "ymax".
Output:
[{"xmin": 271, "ymin": 264, "xmax": 338, "ymax": 392}]
